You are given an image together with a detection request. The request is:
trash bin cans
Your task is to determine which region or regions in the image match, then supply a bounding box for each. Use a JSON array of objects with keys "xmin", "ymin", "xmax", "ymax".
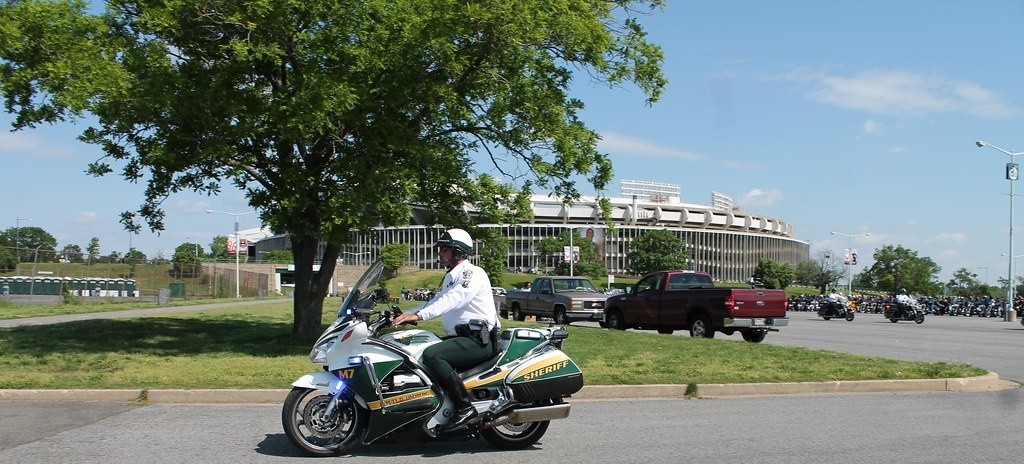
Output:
[
  {"xmin": 258, "ymin": 289, "xmax": 265, "ymax": 298},
  {"xmin": 337, "ymin": 290, "xmax": 342, "ymax": 297},
  {"xmin": 158, "ymin": 288, "xmax": 171, "ymax": 306},
  {"xmin": 1004, "ymin": 302, "xmax": 1010, "ymax": 321}
]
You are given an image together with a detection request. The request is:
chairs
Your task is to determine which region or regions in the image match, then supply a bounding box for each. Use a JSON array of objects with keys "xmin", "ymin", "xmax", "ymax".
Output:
[{"xmin": 545, "ymin": 281, "xmax": 556, "ymax": 290}]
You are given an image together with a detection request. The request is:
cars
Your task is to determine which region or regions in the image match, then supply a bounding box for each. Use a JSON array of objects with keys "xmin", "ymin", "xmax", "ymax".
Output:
[{"xmin": 492, "ymin": 286, "xmax": 507, "ymax": 296}]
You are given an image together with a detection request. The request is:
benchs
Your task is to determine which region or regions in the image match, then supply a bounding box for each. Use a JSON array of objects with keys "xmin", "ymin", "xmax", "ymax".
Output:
[{"xmin": 390, "ymin": 297, "xmax": 401, "ymax": 304}]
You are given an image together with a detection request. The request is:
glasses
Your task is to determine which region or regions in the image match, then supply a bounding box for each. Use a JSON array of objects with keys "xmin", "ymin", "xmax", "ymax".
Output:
[{"xmin": 439, "ymin": 247, "xmax": 448, "ymax": 252}]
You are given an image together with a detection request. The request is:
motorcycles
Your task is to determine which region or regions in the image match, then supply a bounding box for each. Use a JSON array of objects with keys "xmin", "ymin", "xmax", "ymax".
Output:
[
  {"xmin": 788, "ymin": 292, "xmax": 1024, "ymax": 317},
  {"xmin": 817, "ymin": 291, "xmax": 855, "ymax": 321},
  {"xmin": 280, "ymin": 257, "xmax": 584, "ymax": 457},
  {"xmin": 882, "ymin": 294, "xmax": 925, "ymax": 324}
]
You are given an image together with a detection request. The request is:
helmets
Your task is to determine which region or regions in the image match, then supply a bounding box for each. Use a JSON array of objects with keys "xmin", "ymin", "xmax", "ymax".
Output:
[
  {"xmin": 832, "ymin": 288, "xmax": 837, "ymax": 291},
  {"xmin": 436, "ymin": 228, "xmax": 474, "ymax": 256},
  {"xmin": 900, "ymin": 288, "xmax": 906, "ymax": 292}
]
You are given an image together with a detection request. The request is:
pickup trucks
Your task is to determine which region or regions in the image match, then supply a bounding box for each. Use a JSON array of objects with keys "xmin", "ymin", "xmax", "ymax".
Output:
[
  {"xmin": 506, "ymin": 275, "xmax": 610, "ymax": 328},
  {"xmin": 603, "ymin": 268, "xmax": 788, "ymax": 343}
]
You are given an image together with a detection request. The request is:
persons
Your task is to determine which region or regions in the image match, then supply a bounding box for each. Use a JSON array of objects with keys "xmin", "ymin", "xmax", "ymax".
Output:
[
  {"xmin": 790, "ymin": 287, "xmax": 1024, "ymax": 319},
  {"xmin": 585, "ymin": 228, "xmax": 601, "ymax": 260},
  {"xmin": 573, "ymin": 248, "xmax": 579, "ymax": 260},
  {"xmin": 392, "ymin": 228, "xmax": 501, "ymax": 427},
  {"xmin": 520, "ymin": 282, "xmax": 531, "ymax": 292},
  {"xmin": 402, "ymin": 286, "xmax": 437, "ymax": 301}
]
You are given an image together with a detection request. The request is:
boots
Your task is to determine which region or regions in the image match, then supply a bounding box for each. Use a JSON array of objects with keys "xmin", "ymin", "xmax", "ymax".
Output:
[{"xmin": 442, "ymin": 368, "xmax": 479, "ymax": 430}]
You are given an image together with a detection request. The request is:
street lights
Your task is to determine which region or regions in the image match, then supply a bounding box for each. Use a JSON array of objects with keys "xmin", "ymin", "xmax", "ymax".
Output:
[
  {"xmin": 831, "ymin": 232, "xmax": 870, "ymax": 294},
  {"xmin": 977, "ymin": 267, "xmax": 989, "ymax": 286},
  {"xmin": 16, "ymin": 217, "xmax": 32, "ymax": 258},
  {"xmin": 976, "ymin": 140, "xmax": 1024, "ymax": 309},
  {"xmin": 1000, "ymin": 253, "xmax": 1024, "ymax": 299},
  {"xmin": 207, "ymin": 209, "xmax": 265, "ymax": 298}
]
